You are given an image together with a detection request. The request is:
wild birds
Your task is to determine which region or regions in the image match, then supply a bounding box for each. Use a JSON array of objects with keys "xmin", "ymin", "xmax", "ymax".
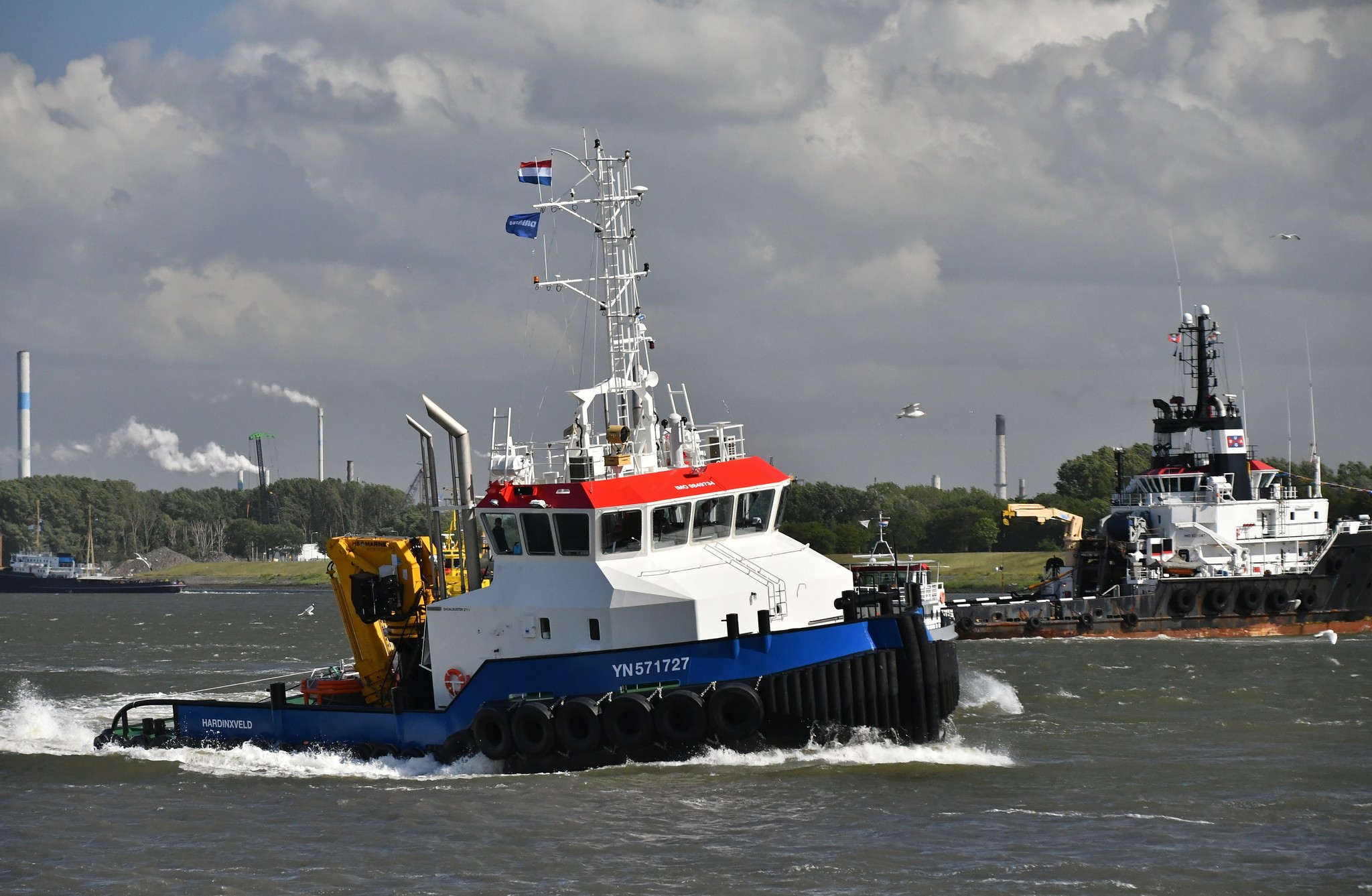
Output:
[
  {"xmin": 1286, "ymin": 598, "xmax": 1301, "ymax": 610},
  {"xmin": 747, "ymin": 591, "xmax": 758, "ymax": 606},
  {"xmin": 895, "ymin": 401, "xmax": 928, "ymax": 421},
  {"xmin": 1312, "ymin": 628, "xmax": 1338, "ymax": 645},
  {"xmin": 1268, "ymin": 233, "xmax": 1302, "ymax": 242},
  {"xmin": 969, "ymin": 410, "xmax": 974, "ymax": 414},
  {"xmin": 297, "ymin": 603, "xmax": 316, "ymax": 618},
  {"xmin": 133, "ymin": 551, "xmax": 153, "ymax": 573}
]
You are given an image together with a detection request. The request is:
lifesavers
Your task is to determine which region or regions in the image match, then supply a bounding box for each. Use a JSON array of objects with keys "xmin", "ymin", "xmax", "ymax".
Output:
[
  {"xmin": 473, "ymin": 705, "xmax": 511, "ymax": 760},
  {"xmin": 1126, "ymin": 613, "xmax": 1139, "ymax": 626},
  {"xmin": 940, "ymin": 591, "xmax": 945, "ymax": 604},
  {"xmin": 93, "ymin": 734, "xmax": 426, "ymax": 762},
  {"xmin": 1296, "ymin": 588, "xmax": 1318, "ymax": 611},
  {"xmin": 551, "ymin": 696, "xmax": 602, "ymax": 763},
  {"xmin": 443, "ymin": 730, "xmax": 477, "ymax": 763},
  {"xmin": 508, "ymin": 702, "xmax": 554, "ymax": 768},
  {"xmin": 1239, "ymin": 585, "xmax": 1263, "ymax": 610},
  {"xmin": 602, "ymin": 692, "xmax": 655, "ymax": 762},
  {"xmin": 1080, "ymin": 612, "xmax": 1095, "ymax": 627},
  {"xmin": 1173, "ymin": 588, "xmax": 1196, "ymax": 613},
  {"xmin": 655, "ymin": 690, "xmax": 705, "ymax": 752},
  {"xmin": 1267, "ymin": 588, "xmax": 1290, "ymax": 610},
  {"xmin": 705, "ymin": 682, "xmax": 765, "ymax": 741},
  {"xmin": 1030, "ymin": 616, "xmax": 1041, "ymax": 629},
  {"xmin": 1206, "ymin": 587, "xmax": 1230, "ymax": 612},
  {"xmin": 960, "ymin": 616, "xmax": 974, "ymax": 632},
  {"xmin": 445, "ymin": 668, "xmax": 465, "ymax": 696}
]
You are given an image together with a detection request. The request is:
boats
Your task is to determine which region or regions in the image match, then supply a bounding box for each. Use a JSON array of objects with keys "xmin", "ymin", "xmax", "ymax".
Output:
[
  {"xmin": 944, "ymin": 303, "xmax": 1372, "ymax": 638},
  {"xmin": 0, "ymin": 501, "xmax": 187, "ymax": 593},
  {"xmin": 92, "ymin": 134, "xmax": 967, "ymax": 779}
]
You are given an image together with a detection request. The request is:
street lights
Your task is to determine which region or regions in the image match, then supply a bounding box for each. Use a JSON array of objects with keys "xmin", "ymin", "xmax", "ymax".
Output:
[
  {"xmin": 391, "ymin": 530, "xmax": 400, "ymax": 537},
  {"xmin": 311, "ymin": 532, "xmax": 318, "ymax": 563}
]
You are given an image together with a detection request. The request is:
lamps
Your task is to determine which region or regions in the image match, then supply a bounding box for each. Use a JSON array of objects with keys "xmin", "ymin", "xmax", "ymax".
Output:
[
  {"xmin": 625, "ymin": 149, "xmax": 631, "ymax": 158},
  {"xmin": 490, "ymin": 499, "xmax": 499, "ymax": 506},
  {"xmin": 528, "ymin": 499, "xmax": 548, "ymax": 509},
  {"xmin": 603, "ymin": 425, "xmax": 632, "ymax": 466},
  {"xmin": 599, "ymin": 301, "xmax": 606, "ymax": 310},
  {"xmin": 635, "ymin": 306, "xmax": 642, "ymax": 315},
  {"xmin": 630, "ymin": 228, "xmax": 637, "ymax": 237},
  {"xmin": 691, "ymin": 464, "xmax": 707, "ymax": 475},
  {"xmin": 1164, "ymin": 443, "xmax": 1172, "ymax": 452},
  {"xmin": 1154, "ymin": 443, "xmax": 1163, "ymax": 453},
  {"xmin": 644, "ymin": 263, "xmax": 650, "ymax": 271},
  {"xmin": 594, "ymin": 223, "xmax": 602, "ymax": 232}
]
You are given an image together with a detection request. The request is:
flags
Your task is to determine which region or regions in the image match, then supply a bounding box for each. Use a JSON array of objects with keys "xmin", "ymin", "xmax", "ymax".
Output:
[
  {"xmin": 517, "ymin": 159, "xmax": 552, "ymax": 187},
  {"xmin": 859, "ymin": 520, "xmax": 871, "ymax": 528},
  {"xmin": 505, "ymin": 212, "xmax": 540, "ymax": 240},
  {"xmin": 429, "ymin": 493, "xmax": 445, "ymax": 502},
  {"xmin": 1207, "ymin": 335, "xmax": 1218, "ymax": 344},
  {"xmin": 878, "ymin": 520, "xmax": 890, "ymax": 527},
  {"xmin": 1168, "ymin": 332, "xmax": 1182, "ymax": 343}
]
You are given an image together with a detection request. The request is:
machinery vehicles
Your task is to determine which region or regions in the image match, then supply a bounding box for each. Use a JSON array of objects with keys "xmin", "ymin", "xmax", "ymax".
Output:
[{"xmin": 247, "ymin": 430, "xmax": 283, "ymax": 524}]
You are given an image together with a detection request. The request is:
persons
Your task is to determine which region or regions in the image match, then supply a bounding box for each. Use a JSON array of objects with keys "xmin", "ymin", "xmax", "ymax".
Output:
[
  {"xmin": 615, "ymin": 512, "xmax": 639, "ymax": 545},
  {"xmin": 493, "ymin": 517, "xmax": 512, "ymax": 552},
  {"xmin": 653, "ymin": 509, "xmax": 674, "ymax": 535},
  {"xmin": 995, "ymin": 565, "xmax": 1004, "ymax": 572}
]
